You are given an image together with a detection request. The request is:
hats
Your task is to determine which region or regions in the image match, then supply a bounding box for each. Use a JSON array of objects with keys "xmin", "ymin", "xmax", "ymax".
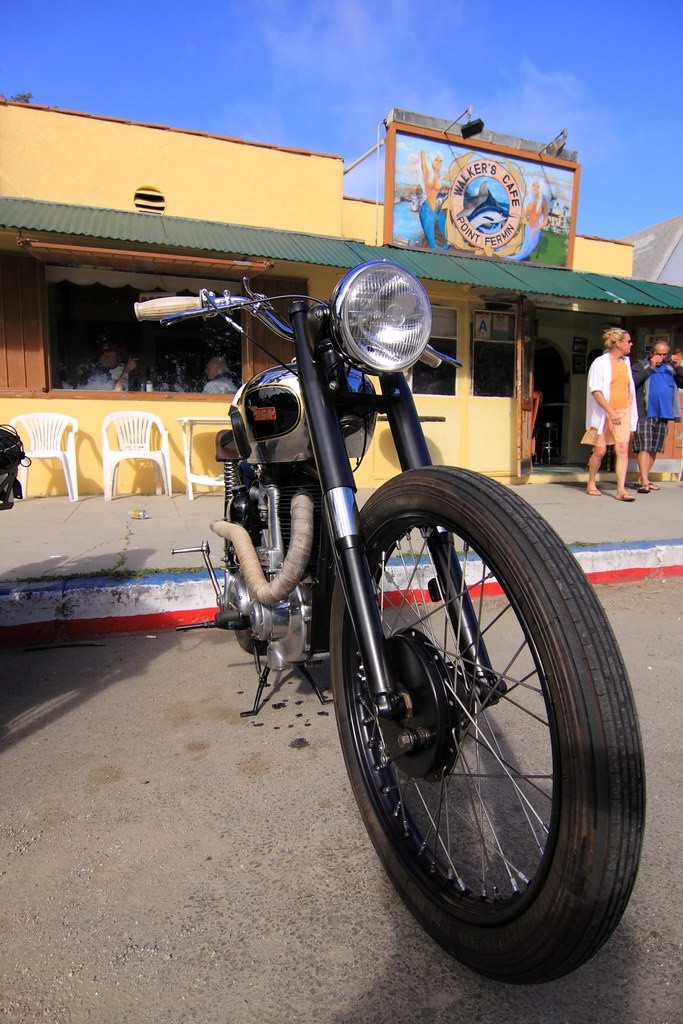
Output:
[{"xmin": 99, "ymin": 343, "xmax": 127, "ymax": 355}]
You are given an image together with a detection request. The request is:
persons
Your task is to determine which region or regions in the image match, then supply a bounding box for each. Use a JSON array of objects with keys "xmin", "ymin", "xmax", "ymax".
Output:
[
  {"xmin": 203, "ymin": 358, "xmax": 237, "ymax": 394},
  {"xmin": 63, "ymin": 346, "xmax": 139, "ymax": 390},
  {"xmin": 582, "ymin": 327, "xmax": 638, "ymax": 502},
  {"xmin": 631, "ymin": 340, "xmax": 683, "ymax": 494}
]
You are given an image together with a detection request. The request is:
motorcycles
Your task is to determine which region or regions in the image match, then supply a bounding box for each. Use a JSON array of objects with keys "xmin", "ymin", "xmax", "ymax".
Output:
[{"xmin": 129, "ymin": 257, "xmax": 650, "ymax": 987}]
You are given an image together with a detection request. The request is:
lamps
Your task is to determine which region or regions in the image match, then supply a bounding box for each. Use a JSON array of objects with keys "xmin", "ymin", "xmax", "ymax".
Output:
[
  {"xmin": 538, "ymin": 129, "xmax": 569, "ymax": 156},
  {"xmin": 443, "ymin": 103, "xmax": 484, "ymax": 139}
]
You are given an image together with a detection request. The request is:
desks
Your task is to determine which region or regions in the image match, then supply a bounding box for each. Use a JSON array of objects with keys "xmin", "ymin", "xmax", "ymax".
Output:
[{"xmin": 175, "ymin": 417, "xmax": 231, "ymax": 501}]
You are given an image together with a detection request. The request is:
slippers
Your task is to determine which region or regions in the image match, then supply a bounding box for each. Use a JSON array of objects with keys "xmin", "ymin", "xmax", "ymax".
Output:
[
  {"xmin": 634, "ymin": 483, "xmax": 660, "ymax": 490},
  {"xmin": 619, "ymin": 494, "xmax": 635, "ymax": 501},
  {"xmin": 637, "ymin": 485, "xmax": 650, "ymax": 492},
  {"xmin": 587, "ymin": 489, "xmax": 602, "ymax": 496}
]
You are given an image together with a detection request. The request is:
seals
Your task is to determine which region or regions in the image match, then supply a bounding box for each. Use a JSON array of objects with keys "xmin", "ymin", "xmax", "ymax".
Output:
[{"xmin": 456, "ymin": 181, "xmax": 489, "ymax": 218}]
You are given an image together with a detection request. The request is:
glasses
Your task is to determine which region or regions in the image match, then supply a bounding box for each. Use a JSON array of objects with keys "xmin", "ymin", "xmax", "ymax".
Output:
[
  {"xmin": 661, "ymin": 358, "xmax": 672, "ymax": 364},
  {"xmin": 621, "ymin": 340, "xmax": 631, "ymax": 344}
]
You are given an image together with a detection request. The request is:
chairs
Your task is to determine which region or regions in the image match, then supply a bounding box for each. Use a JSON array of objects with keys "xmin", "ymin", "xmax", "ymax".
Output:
[
  {"xmin": 100, "ymin": 413, "xmax": 171, "ymax": 500},
  {"xmin": 9, "ymin": 412, "xmax": 78, "ymax": 504},
  {"xmin": 530, "ymin": 393, "xmax": 541, "ymax": 473}
]
